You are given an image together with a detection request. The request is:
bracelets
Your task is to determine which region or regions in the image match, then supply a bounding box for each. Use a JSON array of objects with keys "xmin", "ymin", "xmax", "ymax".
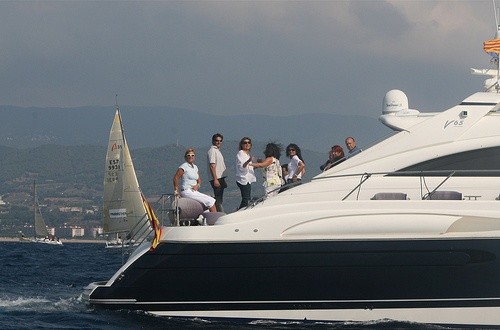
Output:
[
  {"xmin": 197, "ymin": 183, "xmax": 200, "ymax": 188},
  {"xmin": 295, "ymin": 174, "xmax": 298, "ymax": 176}
]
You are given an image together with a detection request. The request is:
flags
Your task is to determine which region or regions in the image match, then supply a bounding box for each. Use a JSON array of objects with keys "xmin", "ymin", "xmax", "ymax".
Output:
[
  {"xmin": 483, "ymin": 38, "xmax": 500, "ymax": 53},
  {"xmin": 141, "ymin": 193, "xmax": 162, "ymax": 251}
]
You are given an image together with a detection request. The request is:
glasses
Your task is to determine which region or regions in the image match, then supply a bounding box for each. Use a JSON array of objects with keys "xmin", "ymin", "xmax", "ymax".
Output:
[
  {"xmin": 242, "ymin": 142, "xmax": 251, "ymax": 144},
  {"xmin": 186, "ymin": 154, "xmax": 195, "ymax": 157}
]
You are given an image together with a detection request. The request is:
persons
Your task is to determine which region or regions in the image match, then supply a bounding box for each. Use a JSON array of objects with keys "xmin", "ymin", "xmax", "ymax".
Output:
[
  {"xmin": 320, "ymin": 145, "xmax": 346, "ymax": 171},
  {"xmin": 207, "ymin": 133, "xmax": 228, "ymax": 213},
  {"xmin": 285, "ymin": 143, "xmax": 305, "ymax": 184},
  {"xmin": 282, "ymin": 164, "xmax": 288, "ymax": 184},
  {"xmin": 234, "ymin": 137, "xmax": 262, "ymax": 210},
  {"xmin": 345, "ymin": 136, "xmax": 359, "ymax": 154},
  {"xmin": 173, "ymin": 147, "xmax": 217, "ymax": 224},
  {"xmin": 248, "ymin": 140, "xmax": 284, "ymax": 200}
]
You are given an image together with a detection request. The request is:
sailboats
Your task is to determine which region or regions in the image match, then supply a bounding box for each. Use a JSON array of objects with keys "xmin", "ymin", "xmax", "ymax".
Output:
[
  {"xmin": 104, "ymin": 106, "xmax": 155, "ymax": 248},
  {"xmin": 26, "ymin": 180, "xmax": 62, "ymax": 244}
]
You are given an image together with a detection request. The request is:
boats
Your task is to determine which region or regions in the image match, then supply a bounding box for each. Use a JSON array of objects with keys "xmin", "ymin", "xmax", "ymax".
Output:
[{"xmin": 81, "ymin": 6, "xmax": 500, "ymax": 328}]
